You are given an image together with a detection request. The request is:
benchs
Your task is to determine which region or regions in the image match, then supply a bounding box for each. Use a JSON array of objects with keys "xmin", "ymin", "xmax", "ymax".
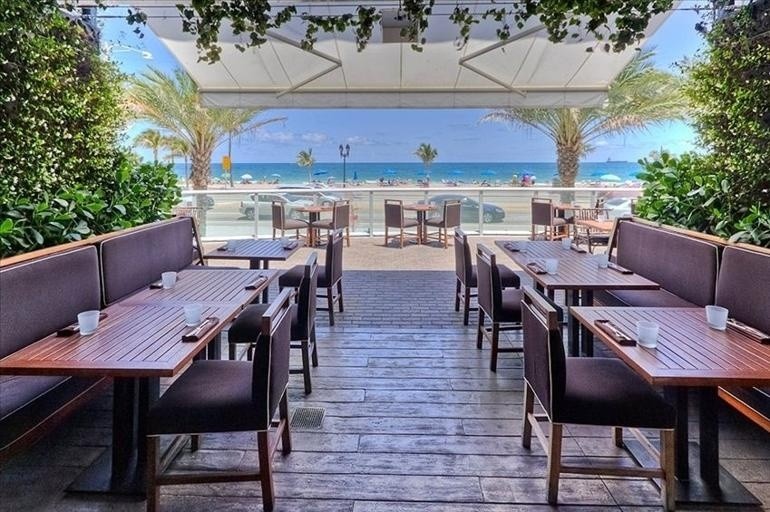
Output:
[
  {"xmin": 594, "ymin": 214, "xmax": 770, "ymax": 434},
  {"xmin": 0, "ymin": 213, "xmax": 246, "ymax": 470}
]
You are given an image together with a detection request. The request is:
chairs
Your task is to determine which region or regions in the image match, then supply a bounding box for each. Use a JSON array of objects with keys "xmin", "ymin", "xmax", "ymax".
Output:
[
  {"xmin": 225, "ymin": 251, "xmax": 322, "ymax": 401},
  {"xmin": 383, "ymin": 199, "xmax": 422, "ymax": 249},
  {"xmin": 473, "ymin": 241, "xmax": 566, "ymax": 376},
  {"xmin": 137, "ymin": 282, "xmax": 299, "ymax": 510},
  {"xmin": 449, "ymin": 226, "xmax": 522, "ymax": 329},
  {"xmin": 270, "ymin": 200, "xmax": 312, "ymax": 248},
  {"xmin": 514, "ymin": 279, "xmax": 684, "ymax": 511},
  {"xmin": 424, "ymin": 199, "xmax": 461, "ymax": 249},
  {"xmin": 312, "ymin": 199, "xmax": 351, "ymax": 249},
  {"xmin": 275, "ymin": 228, "xmax": 348, "ymax": 327},
  {"xmin": 528, "ymin": 194, "xmax": 644, "ymax": 251}
]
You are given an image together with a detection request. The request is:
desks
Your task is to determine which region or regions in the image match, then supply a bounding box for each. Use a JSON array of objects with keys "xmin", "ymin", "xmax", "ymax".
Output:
[
  {"xmin": 565, "ymin": 297, "xmax": 770, "ymax": 506},
  {"xmin": 514, "ymin": 257, "xmax": 663, "ymax": 358},
  {"xmin": 0, "ymin": 303, "xmax": 242, "ymax": 505},
  {"xmin": 497, "ymin": 237, "xmax": 593, "ymax": 300},
  {"xmin": 119, "ymin": 267, "xmax": 280, "ymax": 360},
  {"xmin": 296, "ymin": 205, "xmax": 336, "ymax": 247},
  {"xmin": 402, "ymin": 204, "xmax": 437, "ymax": 245},
  {"xmin": 200, "ymin": 237, "xmax": 305, "ymax": 305}
]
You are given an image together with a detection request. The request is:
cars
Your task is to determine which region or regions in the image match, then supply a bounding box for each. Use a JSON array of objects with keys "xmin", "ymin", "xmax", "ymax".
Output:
[
  {"xmin": 602, "ymin": 182, "xmax": 644, "ymax": 201},
  {"xmin": 600, "ymin": 198, "xmax": 638, "ymax": 220},
  {"xmin": 416, "ymin": 195, "xmax": 506, "ymax": 224},
  {"xmin": 171, "ymin": 193, "xmax": 216, "ymax": 209}
]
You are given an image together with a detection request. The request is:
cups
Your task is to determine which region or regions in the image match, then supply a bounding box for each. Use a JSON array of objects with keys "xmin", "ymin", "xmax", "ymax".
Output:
[
  {"xmin": 636, "ymin": 318, "xmax": 659, "ymax": 348},
  {"xmin": 77, "ymin": 309, "xmax": 99, "ymax": 336},
  {"xmin": 162, "ymin": 271, "xmax": 176, "ymax": 290},
  {"xmin": 227, "ymin": 237, "xmax": 238, "ymax": 252},
  {"xmin": 279, "ymin": 236, "xmax": 289, "ymax": 248},
  {"xmin": 704, "ymin": 303, "xmax": 731, "ymax": 333},
  {"xmin": 184, "ymin": 303, "xmax": 202, "ymax": 327},
  {"xmin": 516, "ymin": 235, "xmax": 609, "ymax": 275}
]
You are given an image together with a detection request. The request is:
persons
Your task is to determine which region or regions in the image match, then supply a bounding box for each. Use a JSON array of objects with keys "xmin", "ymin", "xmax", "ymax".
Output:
[
  {"xmin": 307, "ymin": 175, "xmax": 536, "ymax": 187},
  {"xmin": 195, "ymin": 177, "xmax": 281, "ymax": 184},
  {"xmin": 575, "ymin": 179, "xmax": 654, "ymax": 189}
]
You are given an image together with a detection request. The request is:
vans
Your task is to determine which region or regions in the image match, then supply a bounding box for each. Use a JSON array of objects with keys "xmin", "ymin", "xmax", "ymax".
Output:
[{"xmin": 279, "ymin": 185, "xmax": 343, "ymax": 209}]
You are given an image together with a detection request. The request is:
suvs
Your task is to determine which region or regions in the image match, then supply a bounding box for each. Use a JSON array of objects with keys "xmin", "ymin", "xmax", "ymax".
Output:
[{"xmin": 240, "ymin": 193, "xmax": 305, "ymax": 220}]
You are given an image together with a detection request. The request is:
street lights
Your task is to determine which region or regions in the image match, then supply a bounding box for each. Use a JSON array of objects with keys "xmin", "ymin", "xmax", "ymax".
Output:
[
  {"xmin": 101, "ymin": 42, "xmax": 154, "ymax": 64},
  {"xmin": 338, "ymin": 143, "xmax": 351, "ymax": 188}
]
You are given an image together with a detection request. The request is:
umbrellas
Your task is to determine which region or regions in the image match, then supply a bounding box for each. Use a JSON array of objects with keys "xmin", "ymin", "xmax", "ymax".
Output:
[
  {"xmin": 415, "ymin": 169, "xmax": 433, "ymax": 175},
  {"xmin": 478, "ymin": 167, "xmax": 498, "ymax": 182},
  {"xmin": 519, "ymin": 167, "xmax": 536, "ymax": 176},
  {"xmin": 312, "ymin": 167, "xmax": 329, "ymax": 176},
  {"xmin": 589, "ymin": 167, "xmax": 646, "ymax": 179},
  {"xmin": 221, "ymin": 172, "xmax": 231, "ymax": 179},
  {"xmin": 378, "ymin": 167, "xmax": 399, "ymax": 175},
  {"xmin": 350, "ymin": 166, "xmax": 361, "ymax": 181},
  {"xmin": 448, "ymin": 165, "xmax": 467, "ymax": 180},
  {"xmin": 240, "ymin": 172, "xmax": 252, "ymax": 179},
  {"xmin": 270, "ymin": 173, "xmax": 281, "ymax": 178}
]
